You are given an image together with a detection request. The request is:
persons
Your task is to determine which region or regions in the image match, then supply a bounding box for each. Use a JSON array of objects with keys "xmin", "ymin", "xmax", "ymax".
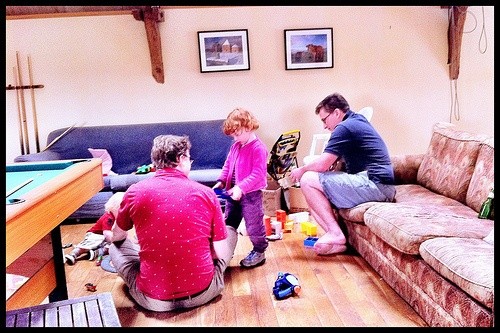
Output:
[
  {"xmin": 108, "ymin": 136, "xmax": 238, "ymax": 311},
  {"xmin": 212, "ymin": 107, "xmax": 269, "ymax": 269},
  {"xmin": 288, "ymin": 93, "xmax": 396, "ymax": 255},
  {"xmin": 65, "ymin": 192, "xmax": 126, "ymax": 265}
]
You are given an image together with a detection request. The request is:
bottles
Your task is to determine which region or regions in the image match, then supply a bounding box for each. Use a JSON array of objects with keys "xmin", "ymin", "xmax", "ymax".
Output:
[{"xmin": 478, "ymin": 188, "xmax": 494, "ymax": 219}]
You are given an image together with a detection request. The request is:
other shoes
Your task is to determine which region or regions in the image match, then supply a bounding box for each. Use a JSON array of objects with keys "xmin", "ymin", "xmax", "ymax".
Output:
[
  {"xmin": 65, "ymin": 253, "xmax": 78, "ymax": 265},
  {"xmin": 87, "ymin": 249, "xmax": 97, "ymax": 260},
  {"xmin": 240, "ymin": 250, "xmax": 266, "ymax": 268}
]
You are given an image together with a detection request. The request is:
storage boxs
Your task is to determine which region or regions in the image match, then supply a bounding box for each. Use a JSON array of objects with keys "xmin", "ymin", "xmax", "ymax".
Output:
[
  {"xmin": 288, "ymin": 183, "xmax": 309, "ymax": 213},
  {"xmin": 263, "ymin": 180, "xmax": 281, "ymax": 217}
]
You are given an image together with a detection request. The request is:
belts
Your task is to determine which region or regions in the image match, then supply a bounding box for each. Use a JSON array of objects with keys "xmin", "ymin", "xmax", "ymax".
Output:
[{"xmin": 163, "ymin": 290, "xmax": 206, "ymax": 302}]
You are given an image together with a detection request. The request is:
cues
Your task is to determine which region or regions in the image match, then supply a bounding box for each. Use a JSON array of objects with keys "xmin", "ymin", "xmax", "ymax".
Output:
[
  {"xmin": 12, "ymin": 51, "xmax": 40, "ymax": 155},
  {"xmin": 5, "ymin": 177, "xmax": 34, "ymax": 198},
  {"xmin": 38, "ymin": 121, "xmax": 78, "ymax": 153}
]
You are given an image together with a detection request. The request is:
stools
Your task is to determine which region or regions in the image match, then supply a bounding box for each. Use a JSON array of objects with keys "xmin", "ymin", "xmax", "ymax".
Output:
[{"xmin": 6, "ymin": 292, "xmax": 121, "ymax": 327}]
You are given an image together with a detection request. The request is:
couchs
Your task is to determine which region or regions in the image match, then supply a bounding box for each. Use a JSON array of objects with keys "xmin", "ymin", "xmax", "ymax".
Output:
[
  {"xmin": 339, "ymin": 121, "xmax": 494, "ymax": 327},
  {"xmin": 14, "ymin": 119, "xmax": 234, "ymax": 219}
]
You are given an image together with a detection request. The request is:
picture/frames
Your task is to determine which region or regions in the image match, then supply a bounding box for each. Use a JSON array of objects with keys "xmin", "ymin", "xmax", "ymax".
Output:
[
  {"xmin": 284, "ymin": 27, "xmax": 334, "ymax": 70},
  {"xmin": 197, "ymin": 29, "xmax": 250, "ymax": 73}
]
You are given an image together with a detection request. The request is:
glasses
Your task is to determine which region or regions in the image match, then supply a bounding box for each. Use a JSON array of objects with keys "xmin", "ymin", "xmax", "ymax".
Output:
[
  {"xmin": 321, "ymin": 112, "xmax": 331, "ymax": 123},
  {"xmin": 183, "ymin": 154, "xmax": 195, "ymax": 164}
]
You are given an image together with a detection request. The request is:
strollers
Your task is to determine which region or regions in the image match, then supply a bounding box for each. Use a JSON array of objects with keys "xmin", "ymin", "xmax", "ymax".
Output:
[{"xmin": 267, "ymin": 129, "xmax": 301, "ymax": 188}]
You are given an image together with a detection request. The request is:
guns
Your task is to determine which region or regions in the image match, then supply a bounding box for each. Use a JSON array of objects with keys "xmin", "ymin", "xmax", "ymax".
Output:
[{"xmin": 273, "ymin": 271, "xmax": 301, "ymax": 300}]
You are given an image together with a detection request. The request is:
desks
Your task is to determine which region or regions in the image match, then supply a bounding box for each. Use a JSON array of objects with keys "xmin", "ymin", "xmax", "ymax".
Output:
[{"xmin": 6, "ymin": 158, "xmax": 104, "ymax": 312}]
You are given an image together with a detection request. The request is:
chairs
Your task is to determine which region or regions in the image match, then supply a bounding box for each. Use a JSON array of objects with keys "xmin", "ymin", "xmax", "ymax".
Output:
[
  {"xmin": 267, "ymin": 129, "xmax": 300, "ymax": 180},
  {"xmin": 303, "ymin": 133, "xmax": 330, "ymax": 165}
]
